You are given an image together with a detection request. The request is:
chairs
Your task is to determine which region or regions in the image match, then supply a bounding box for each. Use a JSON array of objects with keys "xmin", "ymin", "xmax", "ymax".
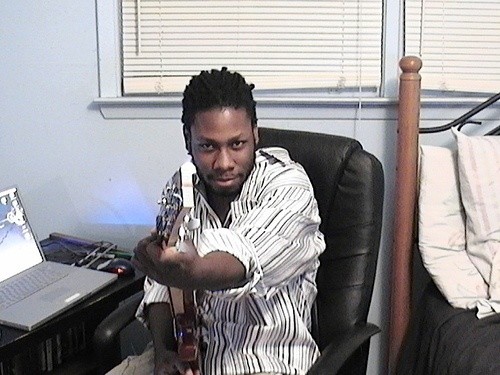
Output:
[{"xmin": 92, "ymin": 130, "xmax": 384, "ymax": 372}]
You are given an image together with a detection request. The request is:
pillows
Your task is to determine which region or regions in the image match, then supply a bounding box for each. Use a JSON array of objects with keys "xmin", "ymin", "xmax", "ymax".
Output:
[
  {"xmin": 419, "ymin": 145, "xmax": 492, "ymax": 311},
  {"xmin": 448, "ymin": 126, "xmax": 499, "ymax": 305}
]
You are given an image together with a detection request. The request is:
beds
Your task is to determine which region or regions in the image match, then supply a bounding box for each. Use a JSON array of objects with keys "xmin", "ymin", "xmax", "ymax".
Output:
[{"xmin": 389, "ymin": 58, "xmax": 498, "ymax": 375}]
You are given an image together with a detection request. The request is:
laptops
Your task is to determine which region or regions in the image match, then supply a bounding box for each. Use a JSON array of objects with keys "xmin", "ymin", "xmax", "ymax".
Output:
[{"xmin": 0, "ymin": 187, "xmax": 117, "ymax": 330}]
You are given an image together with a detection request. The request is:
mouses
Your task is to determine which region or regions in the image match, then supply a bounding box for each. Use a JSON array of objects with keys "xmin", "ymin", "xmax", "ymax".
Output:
[{"xmin": 95, "ymin": 258, "xmax": 136, "ymax": 279}]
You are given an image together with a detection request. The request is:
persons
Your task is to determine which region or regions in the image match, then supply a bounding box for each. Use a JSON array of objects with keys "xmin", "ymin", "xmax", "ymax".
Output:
[{"xmin": 104, "ymin": 67, "xmax": 326, "ymax": 375}]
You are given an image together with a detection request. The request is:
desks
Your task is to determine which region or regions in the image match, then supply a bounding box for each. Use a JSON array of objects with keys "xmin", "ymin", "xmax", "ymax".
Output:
[{"xmin": 0, "ymin": 233, "xmax": 159, "ymax": 374}]
